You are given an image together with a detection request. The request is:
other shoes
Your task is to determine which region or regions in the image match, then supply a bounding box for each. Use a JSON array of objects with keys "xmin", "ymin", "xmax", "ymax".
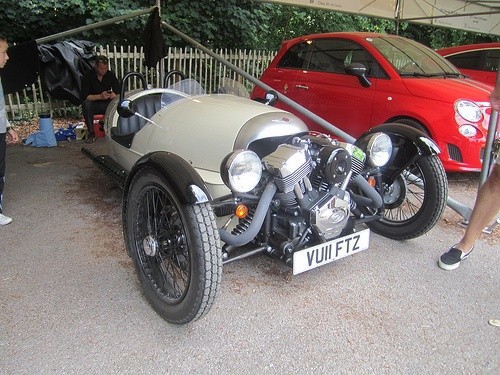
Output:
[
  {"xmin": 0, "ymin": 214, "xmax": 12, "ymax": 226},
  {"xmin": 85, "ymin": 134, "xmax": 96, "ymax": 143}
]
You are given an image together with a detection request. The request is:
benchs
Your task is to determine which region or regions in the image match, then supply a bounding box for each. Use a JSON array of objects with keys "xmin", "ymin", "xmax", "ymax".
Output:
[{"xmin": 111, "ymin": 92, "xmax": 198, "ymax": 149}]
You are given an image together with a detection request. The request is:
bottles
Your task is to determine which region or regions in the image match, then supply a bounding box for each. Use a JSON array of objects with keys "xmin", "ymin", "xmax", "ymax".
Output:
[{"xmin": 38, "ymin": 113, "xmax": 51, "ymax": 131}]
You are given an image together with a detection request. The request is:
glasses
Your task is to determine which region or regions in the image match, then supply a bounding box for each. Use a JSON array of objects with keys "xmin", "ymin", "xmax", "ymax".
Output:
[{"xmin": 99, "ymin": 60, "xmax": 107, "ymax": 63}]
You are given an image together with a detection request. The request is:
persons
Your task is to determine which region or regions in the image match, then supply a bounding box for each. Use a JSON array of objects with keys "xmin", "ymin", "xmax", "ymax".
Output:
[
  {"xmin": 438, "ymin": 68, "xmax": 500, "ymax": 270},
  {"xmin": 0, "ymin": 35, "xmax": 12, "ymax": 226},
  {"xmin": 81, "ymin": 56, "xmax": 121, "ymax": 144}
]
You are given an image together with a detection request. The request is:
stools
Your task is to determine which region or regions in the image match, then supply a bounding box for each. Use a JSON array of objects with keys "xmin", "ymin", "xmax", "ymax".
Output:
[{"xmin": 82, "ymin": 114, "xmax": 107, "ymax": 138}]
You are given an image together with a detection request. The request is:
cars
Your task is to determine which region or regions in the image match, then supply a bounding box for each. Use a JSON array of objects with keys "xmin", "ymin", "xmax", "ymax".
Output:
[
  {"xmin": 434, "ymin": 42, "xmax": 500, "ymax": 88},
  {"xmin": 79, "ymin": 67, "xmax": 450, "ymax": 327},
  {"xmin": 252, "ymin": 31, "xmax": 499, "ymax": 176}
]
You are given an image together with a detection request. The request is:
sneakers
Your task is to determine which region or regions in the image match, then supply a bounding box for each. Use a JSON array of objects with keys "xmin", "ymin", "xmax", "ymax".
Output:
[{"xmin": 439, "ymin": 243, "xmax": 475, "ymax": 271}]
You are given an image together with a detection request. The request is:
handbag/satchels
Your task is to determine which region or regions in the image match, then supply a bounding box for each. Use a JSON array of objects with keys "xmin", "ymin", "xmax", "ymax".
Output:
[{"xmin": 55, "ymin": 128, "xmax": 76, "ymax": 142}]
